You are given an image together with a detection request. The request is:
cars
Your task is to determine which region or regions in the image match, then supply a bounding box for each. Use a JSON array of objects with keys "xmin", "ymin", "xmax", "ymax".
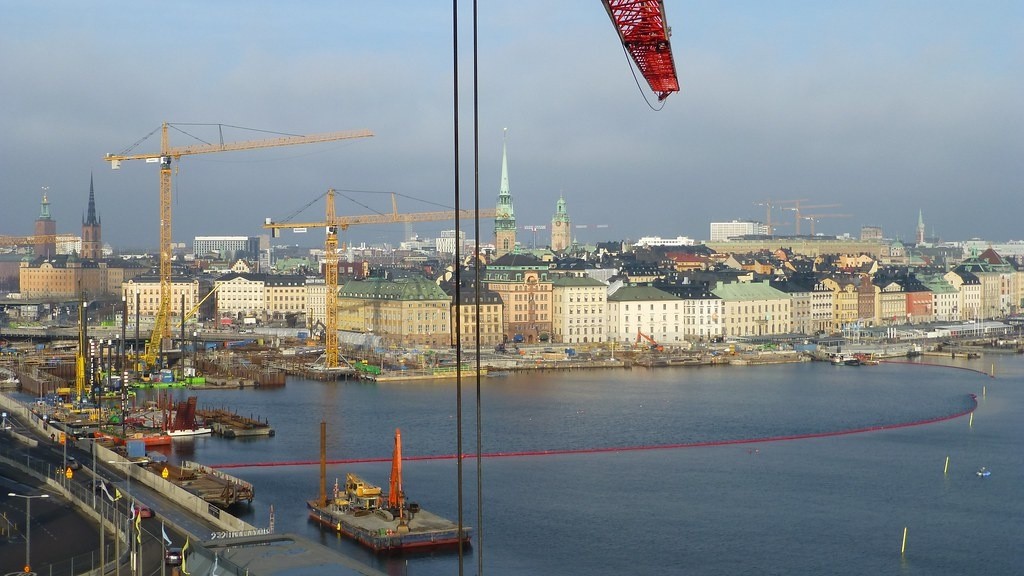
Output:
[
  {"xmin": 55, "ymin": 455, "xmax": 80, "ymax": 473},
  {"xmin": 130, "ymin": 503, "xmax": 151, "ymax": 519},
  {"xmin": 166, "ymin": 548, "xmax": 185, "ymax": 566}
]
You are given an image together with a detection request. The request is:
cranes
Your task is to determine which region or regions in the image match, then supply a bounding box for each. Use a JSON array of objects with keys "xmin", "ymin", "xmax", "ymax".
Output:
[
  {"xmin": 103, "ymin": 120, "xmax": 376, "ymax": 375},
  {"xmin": 260, "ymin": 187, "xmax": 512, "ymax": 367}
]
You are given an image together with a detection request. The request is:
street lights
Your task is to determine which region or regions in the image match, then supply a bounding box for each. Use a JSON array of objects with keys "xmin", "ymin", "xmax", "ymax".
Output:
[
  {"xmin": 7, "ymin": 493, "xmax": 50, "ymax": 571},
  {"xmin": 108, "ymin": 459, "xmax": 148, "ymax": 519},
  {"xmin": 50, "ymin": 420, "xmax": 82, "ymax": 475}
]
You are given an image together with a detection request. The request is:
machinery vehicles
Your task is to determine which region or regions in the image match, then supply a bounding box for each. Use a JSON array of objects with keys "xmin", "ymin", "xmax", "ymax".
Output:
[
  {"xmin": 373, "ymin": 428, "xmax": 411, "ymax": 534},
  {"xmin": 333, "ymin": 472, "xmax": 383, "ymax": 517},
  {"xmin": 634, "ymin": 329, "xmax": 664, "ymax": 351}
]
